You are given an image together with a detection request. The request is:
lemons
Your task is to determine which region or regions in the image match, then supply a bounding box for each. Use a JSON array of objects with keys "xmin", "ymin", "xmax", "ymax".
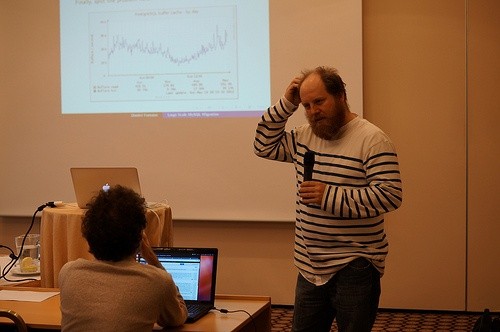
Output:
[{"xmin": 22, "ymin": 257, "xmax": 37, "ymax": 271}]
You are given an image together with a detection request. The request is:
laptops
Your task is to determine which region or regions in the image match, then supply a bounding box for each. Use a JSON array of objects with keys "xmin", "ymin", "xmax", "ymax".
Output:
[
  {"xmin": 135, "ymin": 246, "xmax": 218, "ymax": 323},
  {"xmin": 70, "ymin": 167, "xmax": 146, "ymax": 209}
]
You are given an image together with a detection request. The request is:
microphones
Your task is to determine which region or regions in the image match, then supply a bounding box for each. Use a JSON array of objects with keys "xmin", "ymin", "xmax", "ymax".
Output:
[{"xmin": 303, "ymin": 152, "xmax": 315, "ymax": 182}]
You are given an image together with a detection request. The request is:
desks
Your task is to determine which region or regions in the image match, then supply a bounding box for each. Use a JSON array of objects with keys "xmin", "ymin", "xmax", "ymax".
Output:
[
  {"xmin": 37, "ymin": 202, "xmax": 173, "ymax": 290},
  {"xmin": 0, "ymin": 257, "xmax": 272, "ymax": 332}
]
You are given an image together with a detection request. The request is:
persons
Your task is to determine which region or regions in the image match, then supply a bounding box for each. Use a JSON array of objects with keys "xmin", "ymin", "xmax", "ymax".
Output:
[
  {"xmin": 59, "ymin": 185, "xmax": 188, "ymax": 332},
  {"xmin": 253, "ymin": 66, "xmax": 403, "ymax": 332}
]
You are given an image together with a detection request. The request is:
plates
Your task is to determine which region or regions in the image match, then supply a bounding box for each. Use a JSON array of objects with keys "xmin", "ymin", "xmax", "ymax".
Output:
[{"xmin": 11, "ymin": 268, "xmax": 40, "ymax": 274}]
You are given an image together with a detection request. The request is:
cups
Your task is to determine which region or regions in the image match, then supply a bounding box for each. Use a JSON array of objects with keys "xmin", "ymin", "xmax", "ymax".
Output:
[{"xmin": 15, "ymin": 234, "xmax": 41, "ymax": 273}]
